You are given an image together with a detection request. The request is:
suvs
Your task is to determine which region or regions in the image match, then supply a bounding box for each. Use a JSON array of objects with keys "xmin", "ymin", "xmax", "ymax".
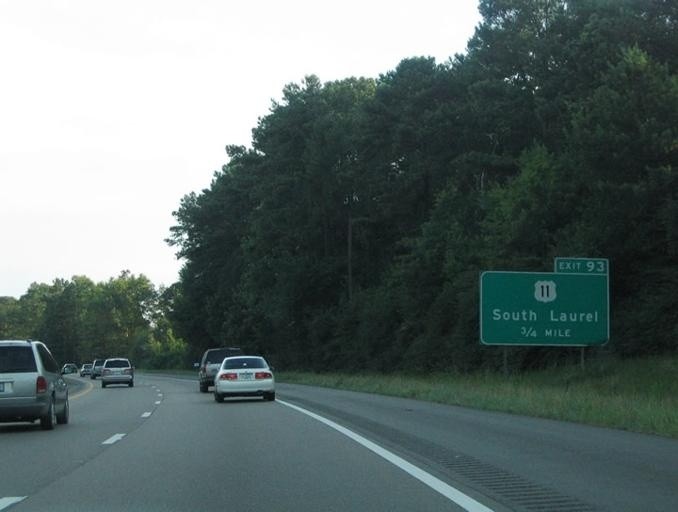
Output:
[
  {"xmin": 91, "ymin": 359, "xmax": 106, "ymax": 379},
  {"xmin": 193, "ymin": 347, "xmax": 247, "ymax": 392}
]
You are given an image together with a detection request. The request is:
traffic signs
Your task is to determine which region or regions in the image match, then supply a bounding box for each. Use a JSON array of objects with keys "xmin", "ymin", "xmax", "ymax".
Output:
[{"xmin": 481, "ymin": 256, "xmax": 611, "ymax": 347}]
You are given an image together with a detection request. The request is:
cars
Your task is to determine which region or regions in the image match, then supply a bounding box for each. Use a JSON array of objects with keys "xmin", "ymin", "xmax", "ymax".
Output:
[
  {"xmin": 214, "ymin": 355, "xmax": 275, "ymax": 402},
  {"xmin": 0, "ymin": 339, "xmax": 70, "ymax": 429},
  {"xmin": 80, "ymin": 364, "xmax": 93, "ymax": 377},
  {"xmin": 102, "ymin": 358, "xmax": 134, "ymax": 388},
  {"xmin": 61, "ymin": 364, "xmax": 78, "ymax": 374}
]
